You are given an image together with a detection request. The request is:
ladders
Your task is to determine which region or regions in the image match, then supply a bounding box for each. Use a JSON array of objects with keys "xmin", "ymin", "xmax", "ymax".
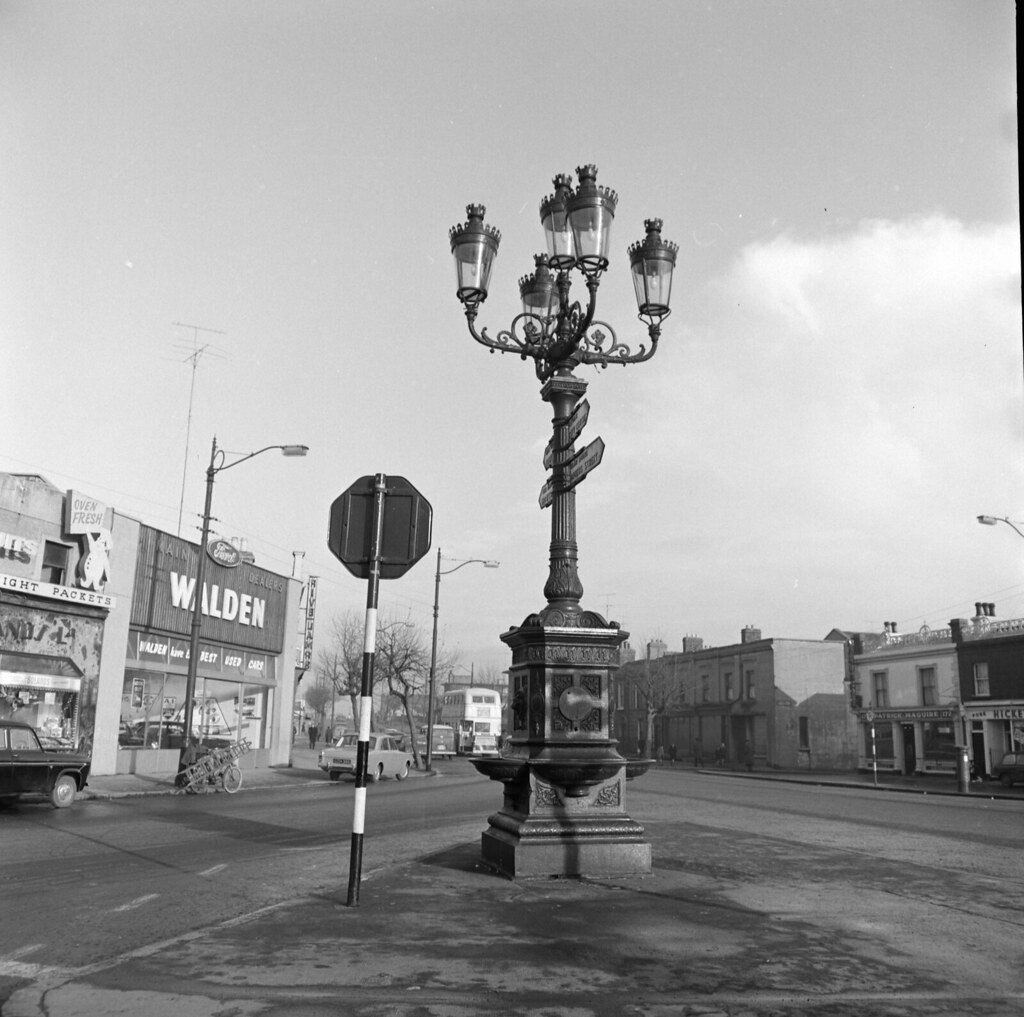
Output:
[{"xmin": 171, "ymin": 737, "xmax": 253, "ymax": 795}]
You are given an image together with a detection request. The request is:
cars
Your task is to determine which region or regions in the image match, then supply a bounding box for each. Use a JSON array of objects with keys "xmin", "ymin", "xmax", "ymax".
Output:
[
  {"xmin": 464, "ymin": 733, "xmax": 499, "ymax": 757},
  {"xmin": 386, "ymin": 729, "xmax": 408, "ymax": 751},
  {"xmin": 0, "ymin": 720, "xmax": 91, "ymax": 810},
  {"xmin": 991, "ymin": 751, "xmax": 1024, "ymax": 787},
  {"xmin": 318, "ymin": 730, "xmax": 415, "ymax": 784},
  {"xmin": 120, "ymin": 721, "xmax": 234, "ymax": 751},
  {"xmin": 415, "ymin": 726, "xmax": 456, "ymax": 762}
]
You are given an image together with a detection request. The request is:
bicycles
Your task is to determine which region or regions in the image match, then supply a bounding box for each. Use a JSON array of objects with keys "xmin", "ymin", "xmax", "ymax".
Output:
[{"xmin": 184, "ymin": 748, "xmax": 243, "ymax": 795}]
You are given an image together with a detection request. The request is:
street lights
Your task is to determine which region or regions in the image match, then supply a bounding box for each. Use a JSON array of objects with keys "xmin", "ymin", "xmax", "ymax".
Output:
[
  {"xmin": 447, "ymin": 164, "xmax": 681, "ymax": 878},
  {"xmin": 427, "ymin": 559, "xmax": 500, "ymax": 770},
  {"xmin": 171, "ymin": 445, "xmax": 311, "ymax": 790}
]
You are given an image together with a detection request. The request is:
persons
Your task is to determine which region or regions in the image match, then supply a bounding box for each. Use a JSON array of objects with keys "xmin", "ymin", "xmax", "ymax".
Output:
[
  {"xmin": 292, "ymin": 722, "xmax": 297, "ymax": 744},
  {"xmin": 308, "ymin": 725, "xmax": 321, "ymax": 750},
  {"xmin": 668, "ymin": 744, "xmax": 677, "ymax": 767},
  {"xmin": 692, "ymin": 738, "xmax": 702, "ymax": 767},
  {"xmin": 716, "ymin": 737, "xmax": 726, "ymax": 769},
  {"xmin": 742, "ymin": 737, "xmax": 756, "ymax": 773},
  {"xmin": 301, "ymin": 722, "xmax": 306, "ymax": 737},
  {"xmin": 325, "ymin": 726, "xmax": 333, "ymax": 747},
  {"xmin": 656, "ymin": 744, "xmax": 664, "ymax": 767},
  {"xmin": 178, "ymin": 739, "xmax": 213, "ymax": 789}
]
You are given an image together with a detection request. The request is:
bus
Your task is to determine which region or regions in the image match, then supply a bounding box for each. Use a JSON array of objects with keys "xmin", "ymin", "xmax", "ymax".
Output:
[{"xmin": 440, "ymin": 689, "xmax": 502, "ymax": 753}]
